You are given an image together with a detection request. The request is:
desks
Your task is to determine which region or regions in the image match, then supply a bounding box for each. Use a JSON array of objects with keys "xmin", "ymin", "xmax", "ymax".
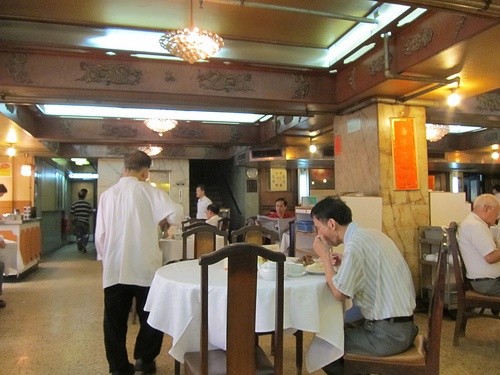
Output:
[{"xmin": 158, "ymin": 231, "xmax": 226, "ymax": 265}]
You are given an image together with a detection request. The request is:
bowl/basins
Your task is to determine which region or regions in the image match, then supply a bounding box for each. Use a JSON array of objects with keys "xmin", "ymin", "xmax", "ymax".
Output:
[{"xmin": 174, "ymin": 233, "xmax": 182, "ymax": 239}]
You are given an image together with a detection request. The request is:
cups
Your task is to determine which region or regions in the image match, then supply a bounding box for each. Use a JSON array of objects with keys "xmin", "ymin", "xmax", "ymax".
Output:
[{"xmin": 291, "ymin": 264, "xmax": 304, "ymax": 273}]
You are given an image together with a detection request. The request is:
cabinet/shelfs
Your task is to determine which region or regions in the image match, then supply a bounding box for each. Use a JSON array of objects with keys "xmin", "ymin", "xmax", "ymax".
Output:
[
  {"xmin": 294, "ymin": 206, "xmax": 322, "ymax": 257},
  {"xmin": 0, "ymin": 216, "xmax": 43, "ymax": 281}
]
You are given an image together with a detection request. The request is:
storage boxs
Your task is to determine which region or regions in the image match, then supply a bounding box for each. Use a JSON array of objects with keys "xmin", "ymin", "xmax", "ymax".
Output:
[{"xmin": 295, "ymin": 220, "xmax": 313, "ymax": 233}]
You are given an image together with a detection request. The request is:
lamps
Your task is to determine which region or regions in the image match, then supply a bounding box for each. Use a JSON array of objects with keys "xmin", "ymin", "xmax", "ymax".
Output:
[
  {"xmin": 158, "ymin": 0, "xmax": 226, "ymax": 66},
  {"xmin": 137, "ymin": 145, "xmax": 163, "ymax": 156},
  {"xmin": 491, "ymin": 143, "xmax": 499, "ymax": 160},
  {"xmin": 427, "ymin": 123, "xmax": 450, "ymax": 143},
  {"xmin": 447, "ymin": 88, "xmax": 461, "ymax": 107},
  {"xmin": 308, "ymin": 138, "xmax": 317, "ymax": 153},
  {"xmin": 6, "ymin": 142, "xmax": 17, "ymax": 156},
  {"xmin": 21, "ymin": 152, "xmax": 31, "ymax": 177},
  {"xmin": 143, "ymin": 117, "xmax": 179, "ymax": 136}
]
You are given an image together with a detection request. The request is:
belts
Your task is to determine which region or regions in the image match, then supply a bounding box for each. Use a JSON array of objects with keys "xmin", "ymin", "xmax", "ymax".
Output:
[{"xmin": 383, "ymin": 315, "xmax": 414, "ymax": 322}]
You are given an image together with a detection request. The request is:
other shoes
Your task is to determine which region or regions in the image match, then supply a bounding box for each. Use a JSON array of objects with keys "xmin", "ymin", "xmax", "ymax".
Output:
[
  {"xmin": 491, "ymin": 305, "xmax": 500, "ymax": 316},
  {"xmin": 82, "ymin": 244, "xmax": 87, "ymax": 252},
  {"xmin": 77, "ymin": 243, "xmax": 81, "ymax": 250},
  {"xmin": 112, "ymin": 363, "xmax": 135, "ymax": 375},
  {"xmin": 0, "ymin": 300, "xmax": 5, "ymax": 308},
  {"xmin": 135, "ymin": 358, "xmax": 156, "ymax": 374}
]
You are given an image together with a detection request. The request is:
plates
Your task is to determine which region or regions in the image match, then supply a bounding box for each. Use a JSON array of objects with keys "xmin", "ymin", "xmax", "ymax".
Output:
[
  {"xmin": 307, "ymin": 263, "xmax": 336, "ymax": 273},
  {"xmin": 287, "ymin": 271, "xmax": 307, "ymax": 276},
  {"xmin": 292, "ymin": 257, "xmax": 316, "ymax": 267}
]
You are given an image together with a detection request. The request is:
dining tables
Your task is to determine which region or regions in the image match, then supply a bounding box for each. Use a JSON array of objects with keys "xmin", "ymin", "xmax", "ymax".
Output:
[{"xmin": 142, "ymin": 255, "xmax": 347, "ymax": 375}]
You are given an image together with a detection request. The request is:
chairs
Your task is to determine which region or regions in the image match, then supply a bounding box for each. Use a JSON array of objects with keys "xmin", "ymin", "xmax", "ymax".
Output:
[
  {"xmin": 182, "ymin": 224, "xmax": 227, "ymax": 260},
  {"xmin": 184, "ymin": 222, "xmax": 210, "ymax": 231},
  {"xmin": 181, "ymin": 217, "xmax": 208, "ymax": 230},
  {"xmin": 230, "ymin": 225, "xmax": 279, "ymax": 245},
  {"xmin": 183, "ymin": 241, "xmax": 290, "ymax": 375},
  {"xmin": 448, "ymin": 219, "xmax": 500, "ymax": 347},
  {"xmin": 217, "ymin": 216, "xmax": 232, "ymax": 231},
  {"xmin": 336, "ymin": 239, "xmax": 451, "ymax": 375}
]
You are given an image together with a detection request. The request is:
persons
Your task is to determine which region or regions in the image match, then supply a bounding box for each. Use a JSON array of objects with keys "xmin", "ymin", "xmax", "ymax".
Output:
[
  {"xmin": 310, "ymin": 195, "xmax": 419, "ymax": 375},
  {"xmin": 456, "ymin": 194, "xmax": 500, "ymax": 296},
  {"xmin": 205, "ymin": 204, "xmax": 224, "ymax": 231},
  {"xmin": 267, "ymin": 198, "xmax": 295, "ymax": 218},
  {"xmin": 195, "ymin": 185, "xmax": 213, "ymax": 220},
  {"xmin": 0, "ymin": 184, "xmax": 8, "ymax": 197},
  {"xmin": 95, "ymin": 150, "xmax": 184, "ymax": 375},
  {"xmin": 69, "ymin": 189, "xmax": 92, "ymax": 253},
  {"xmin": 0, "ymin": 237, "xmax": 6, "ymax": 308},
  {"xmin": 492, "ymin": 185, "xmax": 500, "ymax": 200}
]
